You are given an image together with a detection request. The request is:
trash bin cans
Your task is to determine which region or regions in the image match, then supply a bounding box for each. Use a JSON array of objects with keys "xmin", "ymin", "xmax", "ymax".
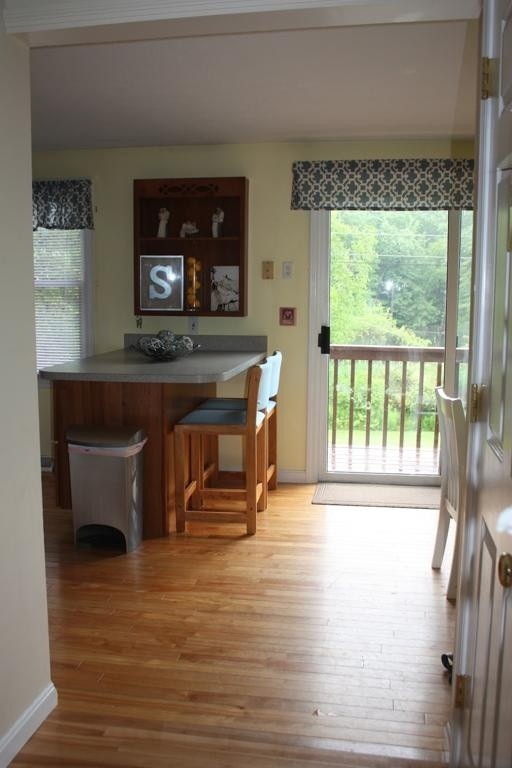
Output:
[{"xmin": 66, "ymin": 428, "xmax": 145, "ymax": 554}]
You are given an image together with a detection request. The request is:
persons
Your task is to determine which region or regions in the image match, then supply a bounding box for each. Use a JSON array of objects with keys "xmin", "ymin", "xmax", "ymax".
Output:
[
  {"xmin": 156, "ymin": 207, "xmax": 170, "ymax": 239},
  {"xmin": 212, "ymin": 205, "xmax": 225, "ymax": 239}
]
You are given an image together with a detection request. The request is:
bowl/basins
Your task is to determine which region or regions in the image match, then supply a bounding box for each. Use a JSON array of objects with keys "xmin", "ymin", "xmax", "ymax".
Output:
[{"xmin": 129, "ymin": 342, "xmax": 200, "ymax": 362}]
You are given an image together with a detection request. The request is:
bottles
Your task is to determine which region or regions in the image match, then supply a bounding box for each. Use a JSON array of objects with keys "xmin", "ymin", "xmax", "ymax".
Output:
[{"xmin": 183, "ymin": 254, "xmax": 203, "ymax": 311}]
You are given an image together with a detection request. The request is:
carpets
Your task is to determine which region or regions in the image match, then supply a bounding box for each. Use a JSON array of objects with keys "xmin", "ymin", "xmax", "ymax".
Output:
[{"xmin": 310, "ymin": 477, "xmax": 443, "ymax": 513}]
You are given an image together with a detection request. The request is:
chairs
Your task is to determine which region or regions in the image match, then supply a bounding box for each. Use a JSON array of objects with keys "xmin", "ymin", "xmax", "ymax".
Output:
[
  {"xmin": 167, "ymin": 358, "xmax": 272, "ymax": 537},
  {"xmin": 428, "ymin": 379, "xmax": 471, "ymax": 608},
  {"xmin": 196, "ymin": 348, "xmax": 283, "ymax": 510}
]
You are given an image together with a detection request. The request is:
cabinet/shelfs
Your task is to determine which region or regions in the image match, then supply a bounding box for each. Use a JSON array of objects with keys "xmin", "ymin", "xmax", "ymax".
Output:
[{"xmin": 129, "ymin": 173, "xmax": 249, "ymax": 320}]
